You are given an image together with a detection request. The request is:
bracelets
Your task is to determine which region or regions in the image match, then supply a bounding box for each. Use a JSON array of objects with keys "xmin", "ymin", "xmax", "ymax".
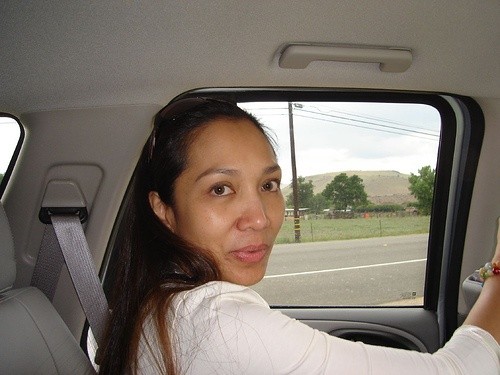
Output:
[{"xmin": 478, "ymin": 262, "xmax": 500, "ymax": 281}]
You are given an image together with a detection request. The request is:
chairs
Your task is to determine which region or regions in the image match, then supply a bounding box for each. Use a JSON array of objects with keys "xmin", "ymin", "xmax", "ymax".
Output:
[{"xmin": 0, "ymin": 201, "xmax": 98, "ymax": 375}]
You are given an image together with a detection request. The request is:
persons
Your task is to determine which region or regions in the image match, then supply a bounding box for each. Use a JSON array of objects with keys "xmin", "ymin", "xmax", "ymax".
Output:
[{"xmin": 86, "ymin": 96, "xmax": 500, "ymax": 375}]
